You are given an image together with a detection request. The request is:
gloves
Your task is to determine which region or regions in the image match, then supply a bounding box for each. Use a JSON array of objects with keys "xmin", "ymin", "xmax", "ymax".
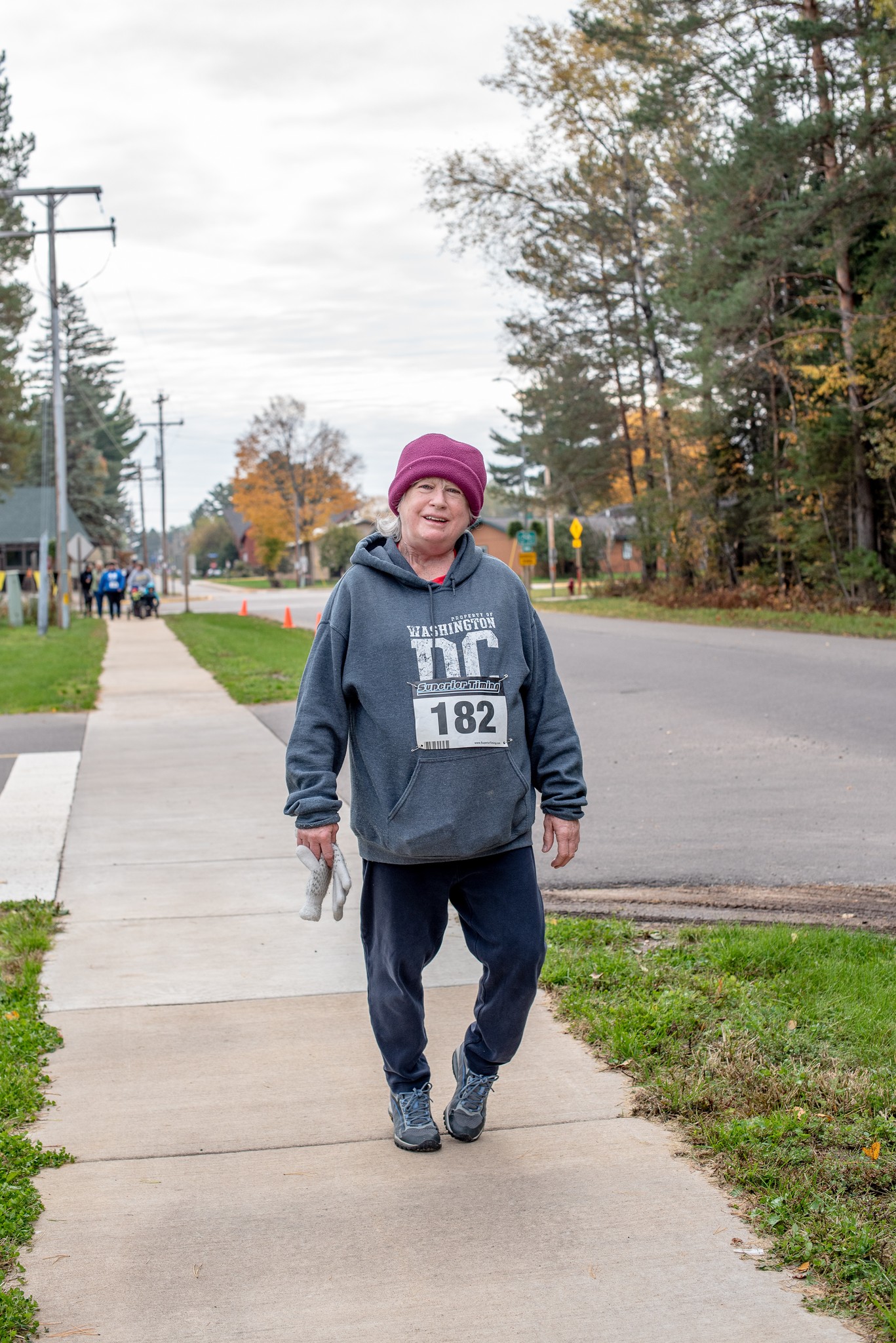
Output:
[{"xmin": 295, "ymin": 841, "xmax": 352, "ymax": 920}]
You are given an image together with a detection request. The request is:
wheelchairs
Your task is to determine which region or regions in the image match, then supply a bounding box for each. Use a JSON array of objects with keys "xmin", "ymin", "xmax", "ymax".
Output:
[{"xmin": 126, "ymin": 593, "xmax": 159, "ymax": 620}]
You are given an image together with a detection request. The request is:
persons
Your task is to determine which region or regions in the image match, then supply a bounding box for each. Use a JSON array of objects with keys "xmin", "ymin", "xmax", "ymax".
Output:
[
  {"xmin": 283, "ymin": 435, "xmax": 587, "ymax": 1151},
  {"xmin": 82, "ymin": 558, "xmax": 160, "ymax": 621}
]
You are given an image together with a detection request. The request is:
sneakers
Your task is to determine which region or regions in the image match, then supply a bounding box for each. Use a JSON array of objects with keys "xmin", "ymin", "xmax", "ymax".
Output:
[
  {"xmin": 388, "ymin": 1082, "xmax": 442, "ymax": 1152},
  {"xmin": 443, "ymin": 1041, "xmax": 499, "ymax": 1141}
]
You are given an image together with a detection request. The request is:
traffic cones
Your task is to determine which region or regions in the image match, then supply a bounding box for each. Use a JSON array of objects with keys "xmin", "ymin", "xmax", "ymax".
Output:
[
  {"xmin": 313, "ymin": 612, "xmax": 322, "ymax": 637},
  {"xmin": 237, "ymin": 598, "xmax": 248, "ymax": 616},
  {"xmin": 281, "ymin": 606, "xmax": 297, "ymax": 628}
]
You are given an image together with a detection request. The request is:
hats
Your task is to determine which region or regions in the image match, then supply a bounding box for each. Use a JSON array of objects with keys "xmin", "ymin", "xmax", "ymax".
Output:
[{"xmin": 388, "ymin": 434, "xmax": 486, "ymax": 527}]
